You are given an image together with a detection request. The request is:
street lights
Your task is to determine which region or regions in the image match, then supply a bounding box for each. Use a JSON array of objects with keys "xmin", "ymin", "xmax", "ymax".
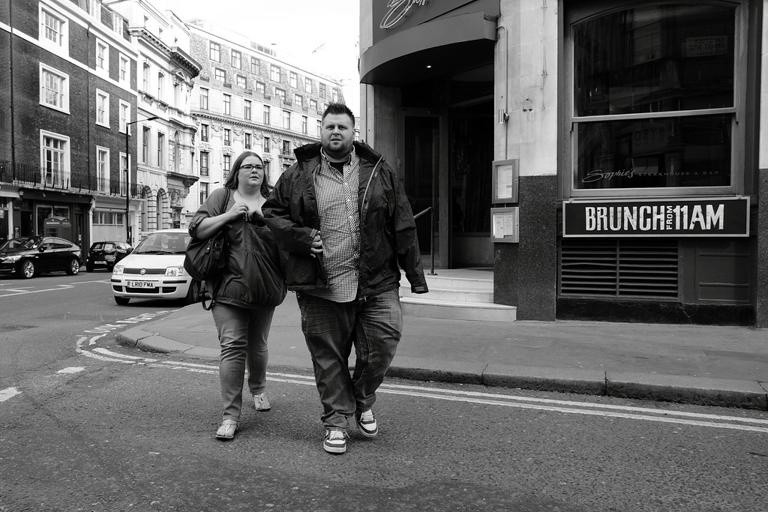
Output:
[{"xmin": 125, "ymin": 116, "xmax": 158, "ymax": 244}]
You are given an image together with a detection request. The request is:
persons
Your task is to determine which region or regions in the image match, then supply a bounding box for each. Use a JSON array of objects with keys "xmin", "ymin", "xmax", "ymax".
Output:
[
  {"xmin": 261, "ymin": 103, "xmax": 428, "ymax": 453},
  {"xmin": 189, "ymin": 151, "xmax": 286, "ymax": 442}
]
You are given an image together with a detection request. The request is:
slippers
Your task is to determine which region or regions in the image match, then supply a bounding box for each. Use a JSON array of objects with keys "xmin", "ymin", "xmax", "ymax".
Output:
[
  {"xmin": 215, "ymin": 421, "xmax": 237, "ymax": 438},
  {"xmin": 254, "ymin": 393, "xmax": 271, "ymax": 411}
]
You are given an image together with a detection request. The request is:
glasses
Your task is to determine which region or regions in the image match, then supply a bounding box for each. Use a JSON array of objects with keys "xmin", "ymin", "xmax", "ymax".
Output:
[{"xmin": 238, "ymin": 164, "xmax": 264, "ymax": 170}]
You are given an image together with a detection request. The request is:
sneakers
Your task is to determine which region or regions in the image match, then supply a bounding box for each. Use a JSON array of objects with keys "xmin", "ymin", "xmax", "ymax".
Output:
[
  {"xmin": 323, "ymin": 428, "xmax": 350, "ymax": 453},
  {"xmin": 356, "ymin": 407, "xmax": 378, "ymax": 438}
]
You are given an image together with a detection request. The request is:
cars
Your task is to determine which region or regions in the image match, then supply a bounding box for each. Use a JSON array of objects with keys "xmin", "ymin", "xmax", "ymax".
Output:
[
  {"xmin": 86, "ymin": 241, "xmax": 132, "ymax": 272},
  {"xmin": 111, "ymin": 231, "xmax": 205, "ymax": 305},
  {"xmin": 0, "ymin": 236, "xmax": 81, "ymax": 278}
]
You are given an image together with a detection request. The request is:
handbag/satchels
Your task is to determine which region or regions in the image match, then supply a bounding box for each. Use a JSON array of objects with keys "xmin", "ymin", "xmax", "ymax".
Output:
[{"xmin": 183, "ymin": 186, "xmax": 231, "ymax": 281}]
